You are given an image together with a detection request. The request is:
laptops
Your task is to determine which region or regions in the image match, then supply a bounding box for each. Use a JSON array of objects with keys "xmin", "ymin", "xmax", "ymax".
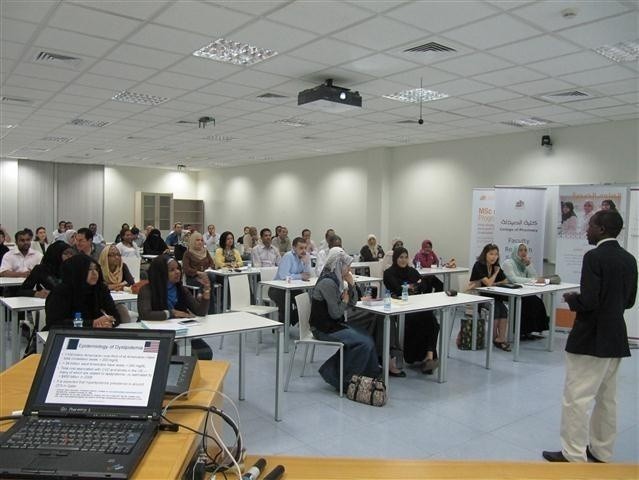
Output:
[{"xmin": 1, "ymin": 326, "xmax": 176, "ymax": 480}]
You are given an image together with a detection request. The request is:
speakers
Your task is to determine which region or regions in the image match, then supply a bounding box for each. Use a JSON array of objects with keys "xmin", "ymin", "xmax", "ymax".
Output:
[{"xmin": 542, "ymin": 135, "xmax": 550, "ymax": 146}]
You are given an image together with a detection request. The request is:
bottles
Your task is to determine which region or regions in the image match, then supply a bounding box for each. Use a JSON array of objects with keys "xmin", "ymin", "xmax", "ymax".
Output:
[
  {"xmin": 402, "ymin": 282, "xmax": 408, "ymax": 303},
  {"xmin": 383, "ymin": 290, "xmax": 391, "ymax": 311},
  {"xmin": 364, "ymin": 289, "xmax": 371, "ymax": 306},
  {"xmin": 73, "ymin": 313, "xmax": 83, "ymax": 328}
]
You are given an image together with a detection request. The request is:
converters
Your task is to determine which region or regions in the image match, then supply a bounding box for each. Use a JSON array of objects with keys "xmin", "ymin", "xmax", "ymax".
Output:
[{"xmin": 187, "ymin": 463, "xmax": 205, "ymax": 480}]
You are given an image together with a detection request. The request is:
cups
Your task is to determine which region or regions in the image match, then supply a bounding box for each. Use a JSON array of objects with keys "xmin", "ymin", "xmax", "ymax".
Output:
[
  {"xmin": 431, "ymin": 264, "xmax": 436, "ymax": 270},
  {"xmin": 544, "ymin": 279, "xmax": 551, "ymax": 285}
]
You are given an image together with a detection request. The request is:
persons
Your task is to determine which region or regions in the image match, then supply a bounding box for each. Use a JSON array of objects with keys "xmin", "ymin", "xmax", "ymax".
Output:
[
  {"xmin": 541, "ymin": 210, "xmax": 639, "ymax": 463},
  {"xmin": 562, "ymin": 202, "xmax": 577, "ymax": 234},
  {"xmin": 0, "ymin": 219, "xmax": 443, "ymax": 394},
  {"xmin": 469, "ymin": 243, "xmax": 513, "ymax": 352},
  {"xmin": 582, "ymin": 200, "xmax": 594, "ymax": 222},
  {"xmin": 501, "ymin": 243, "xmax": 551, "ymax": 336},
  {"xmin": 600, "ymin": 199, "xmax": 619, "ymax": 213}
]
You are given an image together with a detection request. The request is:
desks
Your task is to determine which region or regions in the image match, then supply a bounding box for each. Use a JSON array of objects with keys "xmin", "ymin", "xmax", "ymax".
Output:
[
  {"xmin": 0, "ymin": 350, "xmax": 233, "ymax": 479},
  {"xmin": 202, "ymin": 452, "xmax": 639, "ymax": 480}
]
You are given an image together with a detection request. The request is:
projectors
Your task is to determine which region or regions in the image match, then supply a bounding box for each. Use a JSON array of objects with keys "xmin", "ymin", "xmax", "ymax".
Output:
[{"xmin": 297, "ymin": 78, "xmax": 363, "ymax": 115}]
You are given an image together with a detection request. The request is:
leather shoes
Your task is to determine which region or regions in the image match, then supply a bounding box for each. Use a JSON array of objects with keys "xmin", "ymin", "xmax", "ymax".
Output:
[
  {"xmin": 389, "ymin": 370, "xmax": 406, "ymax": 377},
  {"xmin": 543, "ymin": 451, "xmax": 568, "ymax": 462},
  {"xmin": 586, "ymin": 445, "xmax": 605, "ymax": 463}
]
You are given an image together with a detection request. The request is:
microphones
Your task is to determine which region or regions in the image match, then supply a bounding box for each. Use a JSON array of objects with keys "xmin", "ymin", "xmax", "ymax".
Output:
[
  {"xmin": 238, "ymin": 458, "xmax": 266, "ymax": 480},
  {"xmin": 263, "ymin": 465, "xmax": 285, "ymax": 480}
]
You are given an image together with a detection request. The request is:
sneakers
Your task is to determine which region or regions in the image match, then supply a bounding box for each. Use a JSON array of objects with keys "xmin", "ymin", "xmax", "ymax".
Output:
[{"xmin": 420, "ymin": 359, "xmax": 439, "ymax": 374}]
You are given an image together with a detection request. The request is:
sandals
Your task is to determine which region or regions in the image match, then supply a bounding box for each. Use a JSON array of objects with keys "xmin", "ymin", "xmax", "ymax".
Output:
[{"xmin": 493, "ymin": 341, "xmax": 511, "ymax": 352}]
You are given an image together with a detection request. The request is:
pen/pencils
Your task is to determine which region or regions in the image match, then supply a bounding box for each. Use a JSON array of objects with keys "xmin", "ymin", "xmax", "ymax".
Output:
[
  {"xmin": 100, "ymin": 308, "xmax": 115, "ymax": 325},
  {"xmin": 267, "ymin": 465, "xmax": 285, "ymax": 480},
  {"xmin": 40, "ymin": 284, "xmax": 46, "ymax": 290},
  {"xmin": 241, "ymin": 458, "xmax": 266, "ymax": 480}
]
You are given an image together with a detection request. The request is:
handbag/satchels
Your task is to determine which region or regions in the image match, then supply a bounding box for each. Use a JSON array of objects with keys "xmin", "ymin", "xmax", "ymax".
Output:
[
  {"xmin": 347, "ymin": 374, "xmax": 387, "ymax": 407},
  {"xmin": 456, "ymin": 319, "xmax": 486, "ymax": 350}
]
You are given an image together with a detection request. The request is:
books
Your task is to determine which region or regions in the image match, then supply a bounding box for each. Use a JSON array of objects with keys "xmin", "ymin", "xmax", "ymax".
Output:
[
  {"xmin": 495, "ymin": 281, "xmax": 522, "ymax": 289},
  {"xmin": 526, "ymin": 277, "xmax": 549, "ymax": 288}
]
